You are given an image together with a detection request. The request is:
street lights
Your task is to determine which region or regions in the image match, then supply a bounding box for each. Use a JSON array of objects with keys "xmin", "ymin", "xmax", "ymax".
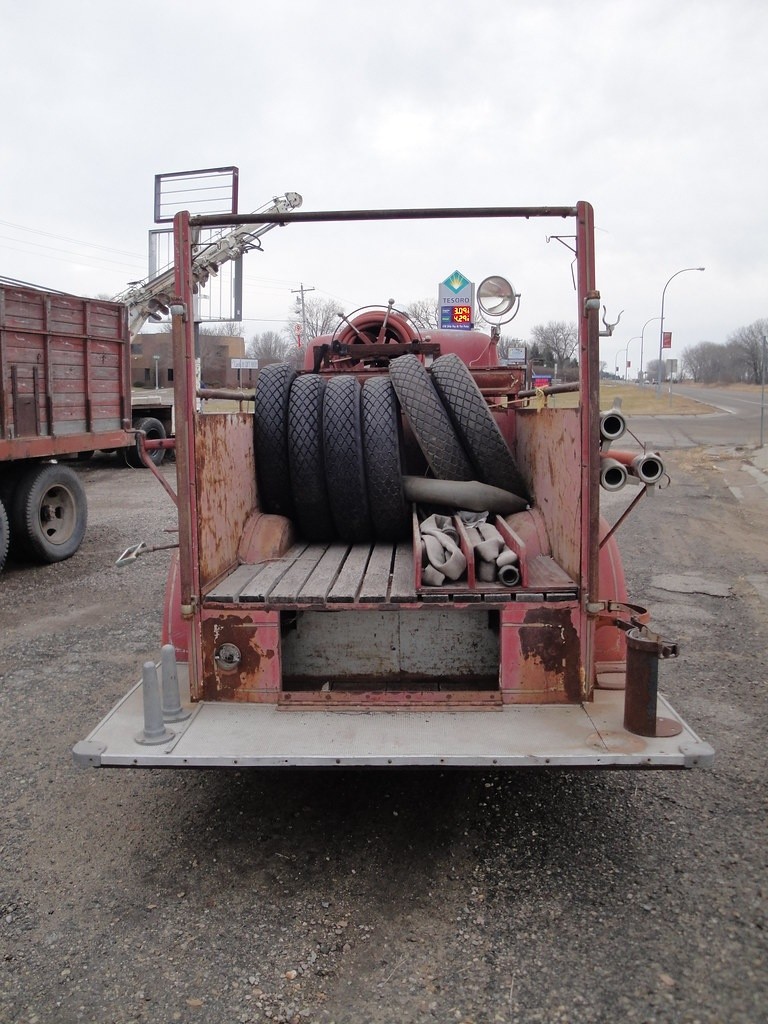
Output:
[
  {"xmin": 637, "ymin": 317, "xmax": 665, "ymax": 387},
  {"xmin": 625, "ymin": 336, "xmax": 643, "ymax": 386},
  {"xmin": 656, "ymin": 267, "xmax": 708, "ymax": 397},
  {"xmin": 614, "ymin": 348, "xmax": 628, "ymax": 384}
]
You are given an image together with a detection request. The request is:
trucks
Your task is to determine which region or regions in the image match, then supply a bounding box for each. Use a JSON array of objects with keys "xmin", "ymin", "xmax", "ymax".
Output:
[
  {"xmin": 0, "ymin": 283, "xmax": 139, "ymax": 569},
  {"xmin": 68, "ymin": 184, "xmax": 715, "ymax": 781}
]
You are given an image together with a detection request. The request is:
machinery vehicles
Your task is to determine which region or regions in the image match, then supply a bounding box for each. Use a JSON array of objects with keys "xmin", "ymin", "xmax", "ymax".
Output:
[{"xmin": 107, "ymin": 191, "xmax": 300, "ymax": 353}]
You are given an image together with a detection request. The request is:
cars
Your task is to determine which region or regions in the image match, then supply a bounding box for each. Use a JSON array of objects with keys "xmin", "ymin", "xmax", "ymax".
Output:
[{"xmin": 634, "ymin": 378, "xmax": 659, "ymax": 386}]
